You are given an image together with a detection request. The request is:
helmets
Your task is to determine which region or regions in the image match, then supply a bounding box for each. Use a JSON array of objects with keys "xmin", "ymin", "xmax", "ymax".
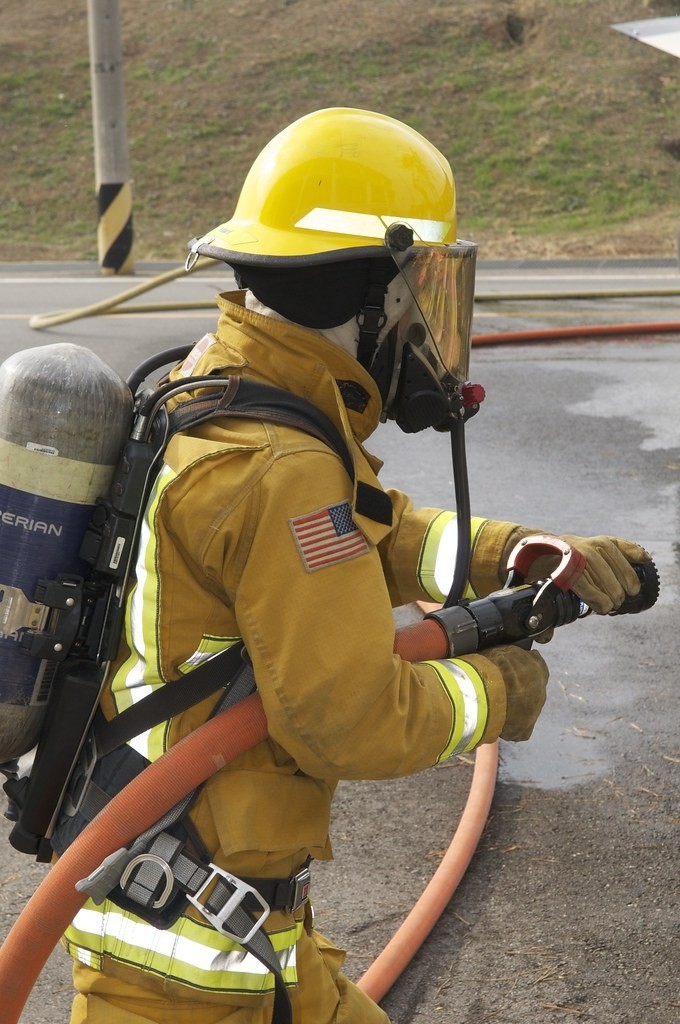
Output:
[{"xmin": 186, "ymin": 107, "xmax": 457, "ymax": 272}]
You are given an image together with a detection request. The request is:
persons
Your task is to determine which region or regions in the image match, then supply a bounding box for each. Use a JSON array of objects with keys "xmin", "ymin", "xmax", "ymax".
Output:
[{"xmin": 0, "ymin": 105, "xmax": 650, "ymax": 1024}]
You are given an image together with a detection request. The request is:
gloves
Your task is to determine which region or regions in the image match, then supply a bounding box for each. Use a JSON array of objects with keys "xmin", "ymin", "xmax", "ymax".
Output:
[
  {"xmin": 479, "ymin": 643, "xmax": 549, "ymax": 743},
  {"xmin": 497, "ymin": 526, "xmax": 653, "ymax": 615}
]
